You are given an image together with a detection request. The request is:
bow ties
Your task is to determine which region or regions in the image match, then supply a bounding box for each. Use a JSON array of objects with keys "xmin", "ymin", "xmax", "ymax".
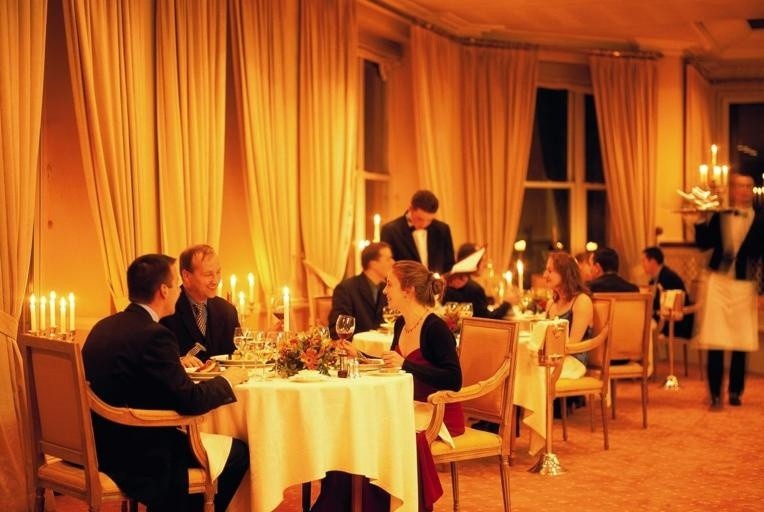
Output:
[{"xmin": 734, "ymin": 210, "xmax": 748, "ymax": 217}]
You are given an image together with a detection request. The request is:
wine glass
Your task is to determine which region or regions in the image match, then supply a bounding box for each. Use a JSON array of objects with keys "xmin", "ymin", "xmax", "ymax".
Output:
[
  {"xmin": 335, "ymin": 314, "xmax": 356, "ymax": 341},
  {"xmin": 383, "ymin": 307, "xmax": 396, "ymax": 336},
  {"xmin": 233, "ymin": 327, "xmax": 290, "ymax": 382},
  {"xmin": 445, "ymin": 301, "xmax": 474, "ymax": 338},
  {"xmin": 270, "ymin": 294, "xmax": 291, "ymax": 329}
]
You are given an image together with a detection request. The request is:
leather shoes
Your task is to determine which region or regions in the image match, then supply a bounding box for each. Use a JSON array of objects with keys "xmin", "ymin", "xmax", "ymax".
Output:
[
  {"xmin": 729, "ymin": 392, "xmax": 741, "ymax": 405},
  {"xmin": 711, "ymin": 396, "xmax": 720, "ymax": 407}
]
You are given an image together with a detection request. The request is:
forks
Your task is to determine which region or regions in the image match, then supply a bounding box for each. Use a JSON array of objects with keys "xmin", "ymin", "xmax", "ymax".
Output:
[{"xmin": 186, "ymin": 346, "xmax": 203, "ymax": 355}]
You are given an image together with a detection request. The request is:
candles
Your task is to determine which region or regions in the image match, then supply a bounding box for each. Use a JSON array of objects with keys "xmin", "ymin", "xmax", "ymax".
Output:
[
  {"xmin": 232, "ymin": 286, "xmax": 236, "ymax": 304},
  {"xmin": 70, "ymin": 303, "xmax": 75, "ymax": 330},
  {"xmin": 712, "ymin": 151, "xmax": 717, "ymax": 166},
  {"xmin": 704, "ymin": 172, "xmax": 708, "ymax": 185},
  {"xmin": 249, "ymin": 281, "xmax": 255, "ymax": 304},
  {"xmin": 49, "ymin": 299, "xmax": 56, "ymax": 326},
  {"xmin": 700, "ymin": 171, "xmax": 704, "ymax": 184},
  {"xmin": 29, "ymin": 303, "xmax": 37, "ymax": 331},
  {"xmin": 518, "ymin": 271, "xmax": 523, "ymax": 292},
  {"xmin": 40, "ymin": 304, "xmax": 46, "ymax": 331},
  {"xmin": 713, "ymin": 167, "xmax": 721, "ymax": 186},
  {"xmin": 375, "ymin": 224, "xmax": 380, "ymax": 244},
  {"xmin": 60, "ymin": 307, "xmax": 67, "ymax": 332},
  {"xmin": 284, "ymin": 295, "xmax": 290, "ymax": 332},
  {"xmin": 216, "ymin": 286, "xmax": 222, "ymax": 296},
  {"xmin": 240, "ymin": 297, "xmax": 245, "ymax": 309},
  {"xmin": 722, "ymin": 172, "xmax": 728, "ymax": 185}
]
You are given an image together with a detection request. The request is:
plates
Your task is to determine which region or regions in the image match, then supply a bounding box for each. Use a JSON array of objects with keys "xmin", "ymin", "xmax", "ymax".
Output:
[
  {"xmin": 184, "ymin": 354, "xmax": 407, "ymax": 376},
  {"xmin": 381, "ymin": 324, "xmax": 395, "ymax": 329}
]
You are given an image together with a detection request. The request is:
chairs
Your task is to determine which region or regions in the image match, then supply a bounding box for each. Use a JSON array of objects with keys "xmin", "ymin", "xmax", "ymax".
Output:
[
  {"xmin": 653, "ymin": 279, "xmax": 707, "ymax": 386},
  {"xmin": 554, "ymin": 297, "xmax": 616, "ymax": 451},
  {"xmin": 426, "ymin": 317, "xmax": 520, "ymax": 512},
  {"xmin": 591, "ymin": 293, "xmax": 654, "ymax": 429},
  {"xmin": 20, "ymin": 331, "xmax": 220, "ymax": 512}
]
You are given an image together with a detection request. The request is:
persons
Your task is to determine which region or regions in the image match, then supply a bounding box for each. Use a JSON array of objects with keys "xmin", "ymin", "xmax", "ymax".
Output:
[
  {"xmin": 327, "ymin": 242, "xmax": 397, "ymax": 341},
  {"xmin": 159, "ymin": 245, "xmax": 244, "ymax": 368},
  {"xmin": 310, "ymin": 261, "xmax": 464, "ymax": 512},
  {"xmin": 81, "ymin": 253, "xmax": 248, "ymax": 512},
  {"xmin": 380, "ymin": 189, "xmax": 469, "ymax": 300},
  {"xmin": 694, "ymin": 173, "xmax": 764, "ymax": 412},
  {"xmin": 642, "ymin": 248, "xmax": 694, "ymax": 339},
  {"xmin": 437, "ymin": 244, "xmax": 640, "ymax": 435}
]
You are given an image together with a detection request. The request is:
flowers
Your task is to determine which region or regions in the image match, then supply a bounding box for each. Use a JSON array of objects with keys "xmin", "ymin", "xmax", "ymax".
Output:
[{"xmin": 271, "ymin": 325, "xmax": 338, "ymax": 379}]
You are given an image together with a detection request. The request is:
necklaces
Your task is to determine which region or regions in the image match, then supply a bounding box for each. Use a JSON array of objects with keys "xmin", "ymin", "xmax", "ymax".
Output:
[{"xmin": 404, "ymin": 309, "xmax": 428, "ymax": 333}]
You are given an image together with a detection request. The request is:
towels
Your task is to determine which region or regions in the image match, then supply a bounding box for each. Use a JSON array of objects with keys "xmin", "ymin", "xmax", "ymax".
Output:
[
  {"xmin": 665, "ymin": 289, "xmax": 684, "ymax": 308},
  {"xmin": 177, "ymin": 429, "xmax": 234, "ymax": 483},
  {"xmin": 560, "ymin": 353, "xmax": 588, "ymax": 380},
  {"xmin": 414, "ymin": 400, "xmax": 455, "ymax": 449},
  {"xmin": 529, "ymin": 317, "xmax": 571, "ymax": 351}
]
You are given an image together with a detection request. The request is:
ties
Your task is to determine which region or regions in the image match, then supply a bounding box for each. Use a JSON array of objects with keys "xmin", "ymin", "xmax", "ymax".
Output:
[{"xmin": 192, "ymin": 303, "xmax": 206, "ymax": 337}]
